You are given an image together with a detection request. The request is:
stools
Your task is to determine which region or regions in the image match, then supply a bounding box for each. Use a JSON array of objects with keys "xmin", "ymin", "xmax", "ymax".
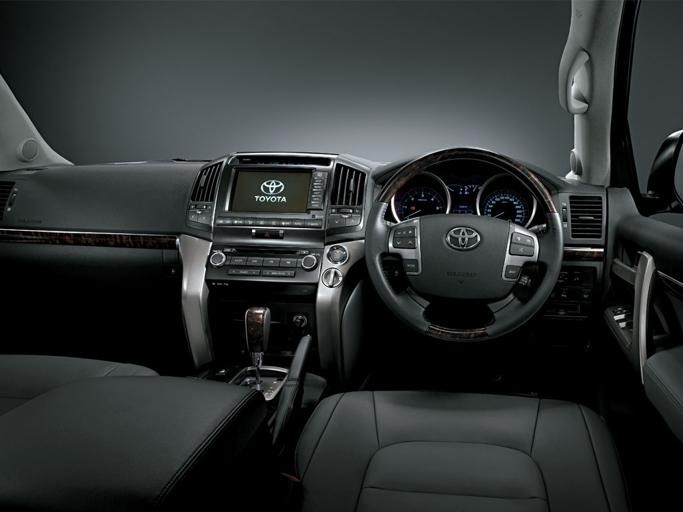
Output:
[
  {"xmin": 0, "ymin": 346, "xmax": 163, "ymax": 417},
  {"xmin": 294, "ymin": 385, "xmax": 632, "ymax": 511}
]
